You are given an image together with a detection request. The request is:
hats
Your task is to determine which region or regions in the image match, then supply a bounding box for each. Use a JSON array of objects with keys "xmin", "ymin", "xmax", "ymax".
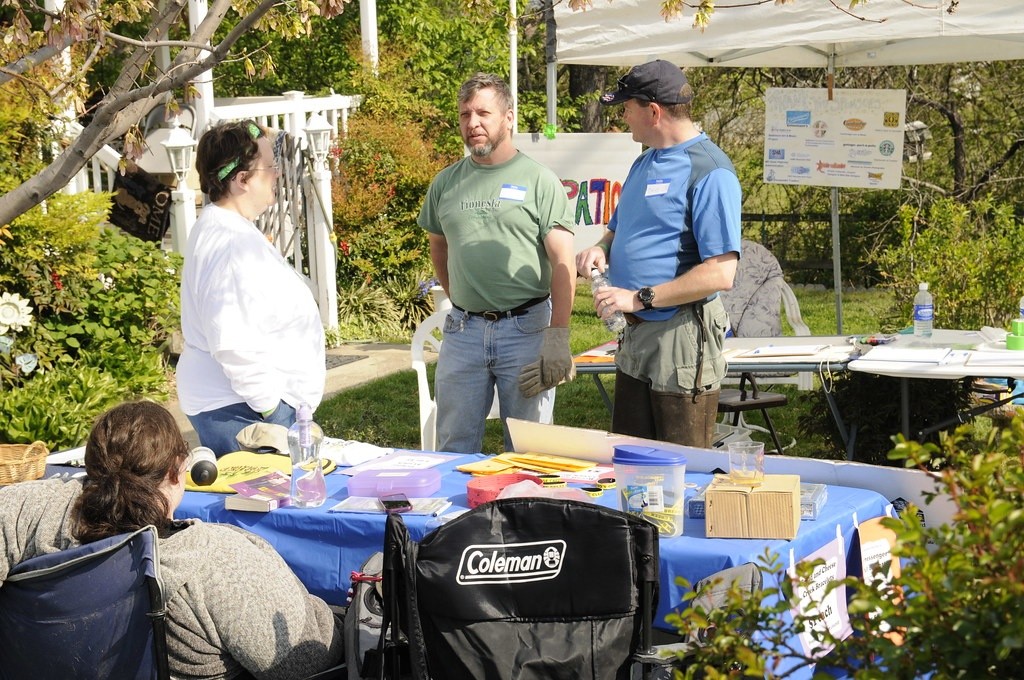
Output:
[{"xmin": 598, "ymin": 61, "xmax": 694, "ymax": 106}]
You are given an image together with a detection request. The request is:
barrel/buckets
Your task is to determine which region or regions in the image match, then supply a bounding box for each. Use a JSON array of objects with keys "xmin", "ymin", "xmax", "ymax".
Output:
[{"xmin": 612, "ymin": 445, "xmax": 686, "ymax": 539}]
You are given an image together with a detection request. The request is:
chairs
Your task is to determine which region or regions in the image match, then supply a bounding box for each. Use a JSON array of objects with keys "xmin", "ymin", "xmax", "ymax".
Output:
[
  {"xmin": 345, "ymin": 497, "xmax": 762, "ymax": 680},
  {"xmin": 719, "ymin": 240, "xmax": 816, "ymax": 452},
  {"xmin": 410, "ymin": 307, "xmax": 577, "ymax": 457},
  {"xmin": 0, "ymin": 524, "xmax": 167, "ymax": 680},
  {"xmin": 137, "ymin": 101, "xmax": 198, "ymax": 176}
]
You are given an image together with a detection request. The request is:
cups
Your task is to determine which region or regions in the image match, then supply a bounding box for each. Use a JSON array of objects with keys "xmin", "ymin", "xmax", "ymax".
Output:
[{"xmin": 728, "ymin": 441, "xmax": 765, "ymax": 486}]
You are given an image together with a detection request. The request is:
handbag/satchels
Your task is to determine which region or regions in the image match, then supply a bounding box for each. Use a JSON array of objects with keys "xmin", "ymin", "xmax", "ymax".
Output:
[{"xmin": 108, "ymin": 164, "xmax": 173, "ymax": 250}]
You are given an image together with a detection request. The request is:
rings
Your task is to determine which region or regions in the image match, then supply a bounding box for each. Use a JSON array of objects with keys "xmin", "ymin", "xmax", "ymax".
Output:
[{"xmin": 604, "ymin": 298, "xmax": 610, "ymax": 307}]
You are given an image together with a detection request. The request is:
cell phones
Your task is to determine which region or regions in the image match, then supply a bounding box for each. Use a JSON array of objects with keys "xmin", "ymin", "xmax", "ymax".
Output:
[{"xmin": 378, "ymin": 494, "xmax": 413, "ymax": 514}]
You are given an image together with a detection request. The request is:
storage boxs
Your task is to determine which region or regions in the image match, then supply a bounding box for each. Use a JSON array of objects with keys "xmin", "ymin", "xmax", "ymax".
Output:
[
  {"xmin": 345, "ymin": 468, "xmax": 442, "ymax": 498},
  {"xmin": 611, "ymin": 442, "xmax": 685, "ymax": 538},
  {"xmin": 702, "ymin": 473, "xmax": 801, "ymax": 541}
]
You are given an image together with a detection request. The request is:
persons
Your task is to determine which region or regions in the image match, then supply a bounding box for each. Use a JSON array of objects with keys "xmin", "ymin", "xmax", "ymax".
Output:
[
  {"xmin": 576, "ymin": 59, "xmax": 741, "ymax": 450},
  {"xmin": 0, "ymin": 401, "xmax": 347, "ymax": 680},
  {"xmin": 417, "ymin": 74, "xmax": 577, "ymax": 454},
  {"xmin": 175, "ymin": 119, "xmax": 325, "ymax": 460}
]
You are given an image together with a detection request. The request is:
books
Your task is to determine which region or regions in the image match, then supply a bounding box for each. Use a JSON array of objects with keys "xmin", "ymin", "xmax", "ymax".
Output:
[{"xmin": 225, "ymin": 470, "xmax": 291, "ymax": 512}]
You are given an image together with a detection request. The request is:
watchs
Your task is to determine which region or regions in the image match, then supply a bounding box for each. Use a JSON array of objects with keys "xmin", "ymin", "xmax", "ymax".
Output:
[{"xmin": 637, "ymin": 287, "xmax": 655, "ymax": 309}]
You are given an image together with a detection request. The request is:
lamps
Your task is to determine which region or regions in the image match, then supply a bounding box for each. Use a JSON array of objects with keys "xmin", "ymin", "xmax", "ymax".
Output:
[
  {"xmin": 159, "ymin": 115, "xmax": 198, "ymax": 191},
  {"xmin": 302, "ymin": 108, "xmax": 334, "ymax": 174}
]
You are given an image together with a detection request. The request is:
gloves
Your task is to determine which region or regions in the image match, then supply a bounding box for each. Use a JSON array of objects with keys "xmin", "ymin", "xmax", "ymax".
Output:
[
  {"xmin": 517, "ymin": 346, "xmax": 577, "ymax": 397},
  {"xmin": 539, "ymin": 325, "xmax": 574, "ymax": 387}
]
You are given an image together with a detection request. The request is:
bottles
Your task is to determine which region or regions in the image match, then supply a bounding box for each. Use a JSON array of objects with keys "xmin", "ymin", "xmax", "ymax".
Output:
[
  {"xmin": 1019, "ymin": 296, "xmax": 1024, "ymax": 322},
  {"xmin": 287, "ymin": 402, "xmax": 327, "ymax": 510},
  {"xmin": 189, "ymin": 445, "xmax": 218, "ymax": 486},
  {"xmin": 589, "ymin": 269, "xmax": 626, "ymax": 332},
  {"xmin": 913, "ymin": 282, "xmax": 933, "ymax": 339}
]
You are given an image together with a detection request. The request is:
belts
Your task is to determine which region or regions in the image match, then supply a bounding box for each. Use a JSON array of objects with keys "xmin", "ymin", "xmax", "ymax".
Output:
[
  {"xmin": 623, "ymin": 312, "xmax": 646, "ymax": 326},
  {"xmin": 453, "ymin": 294, "xmax": 549, "ymax": 323}
]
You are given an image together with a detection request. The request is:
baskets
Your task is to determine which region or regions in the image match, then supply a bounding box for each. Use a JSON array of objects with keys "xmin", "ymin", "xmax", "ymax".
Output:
[{"xmin": 1, "ymin": 440, "xmax": 50, "ymax": 485}]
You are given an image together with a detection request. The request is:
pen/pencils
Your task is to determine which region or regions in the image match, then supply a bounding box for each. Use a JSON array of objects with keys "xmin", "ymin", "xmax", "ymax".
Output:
[
  {"xmin": 753, "ymin": 344, "xmax": 773, "ymax": 354},
  {"xmin": 846, "ymin": 336, "xmax": 898, "ymax": 344}
]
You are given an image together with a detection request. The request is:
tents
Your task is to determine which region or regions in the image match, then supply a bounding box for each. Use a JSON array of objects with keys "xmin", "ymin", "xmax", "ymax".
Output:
[{"xmin": 545, "ymin": 0, "xmax": 1024, "ymax": 380}]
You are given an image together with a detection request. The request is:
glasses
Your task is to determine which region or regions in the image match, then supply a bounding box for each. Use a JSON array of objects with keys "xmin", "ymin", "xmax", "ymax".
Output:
[
  {"xmin": 218, "ymin": 120, "xmax": 264, "ymax": 182},
  {"xmin": 619, "ymin": 72, "xmax": 658, "ymax": 103}
]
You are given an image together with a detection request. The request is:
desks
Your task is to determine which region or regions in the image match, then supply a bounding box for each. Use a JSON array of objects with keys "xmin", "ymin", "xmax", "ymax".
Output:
[
  {"xmin": 0, "ymin": 428, "xmax": 963, "ymax": 680},
  {"xmin": 848, "ymin": 326, "xmax": 1024, "ymax": 469},
  {"xmin": 569, "ymin": 327, "xmax": 878, "ymax": 464}
]
